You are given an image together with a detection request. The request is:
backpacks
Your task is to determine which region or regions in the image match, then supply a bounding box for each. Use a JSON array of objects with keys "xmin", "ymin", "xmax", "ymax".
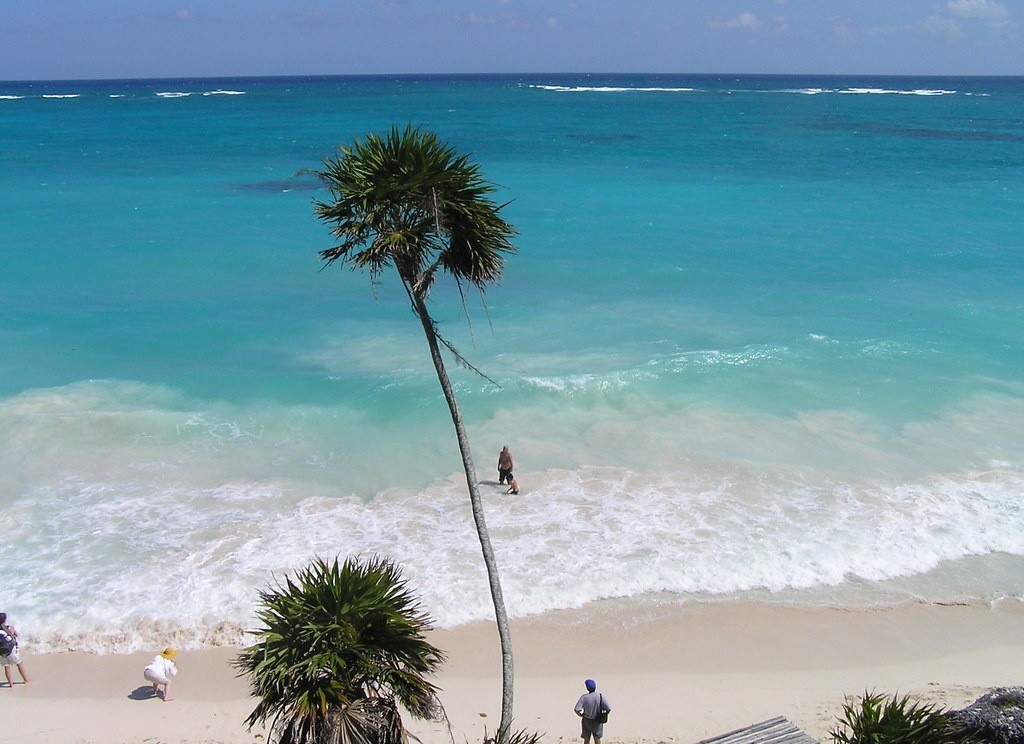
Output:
[{"xmin": 0, "ymin": 626, "xmax": 15, "ymax": 658}]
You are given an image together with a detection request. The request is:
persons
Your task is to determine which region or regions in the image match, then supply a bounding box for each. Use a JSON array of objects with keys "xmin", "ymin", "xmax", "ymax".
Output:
[
  {"xmin": 573, "ymin": 679, "xmax": 612, "ymax": 744},
  {"xmin": 0, "ymin": 612, "xmax": 32, "ymax": 688},
  {"xmin": 505, "ymin": 474, "xmax": 520, "ymax": 494},
  {"xmin": 498, "ymin": 446, "xmax": 513, "ymax": 485},
  {"xmin": 144, "ymin": 646, "xmax": 178, "ymax": 701}
]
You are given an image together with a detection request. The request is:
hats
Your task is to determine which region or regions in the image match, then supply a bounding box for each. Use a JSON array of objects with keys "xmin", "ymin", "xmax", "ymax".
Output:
[
  {"xmin": 585, "ymin": 679, "xmax": 596, "ymax": 690},
  {"xmin": 160, "ymin": 648, "xmax": 177, "ymax": 660}
]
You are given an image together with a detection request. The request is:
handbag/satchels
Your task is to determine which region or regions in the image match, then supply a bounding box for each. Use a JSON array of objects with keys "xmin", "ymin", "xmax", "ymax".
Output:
[{"xmin": 597, "ymin": 713, "xmax": 608, "ymax": 723}]
